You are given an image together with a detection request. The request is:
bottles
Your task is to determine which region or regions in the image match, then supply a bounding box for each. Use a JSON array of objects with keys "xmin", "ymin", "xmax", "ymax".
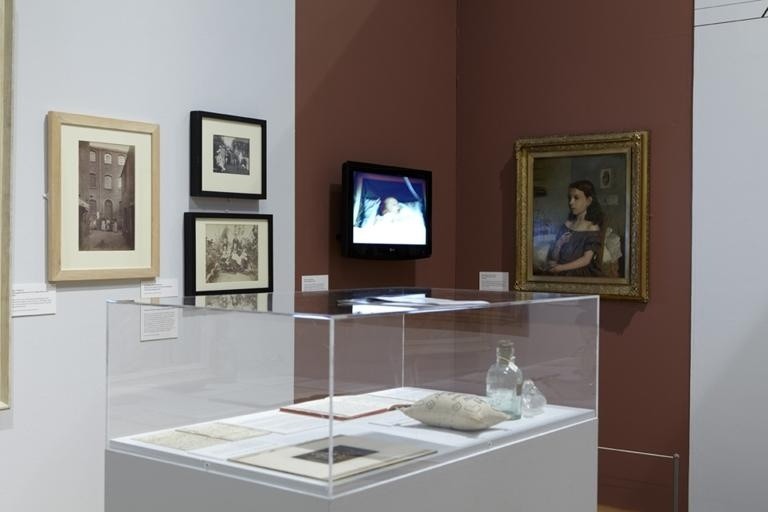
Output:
[{"xmin": 488, "ymin": 338, "xmax": 523, "ymax": 419}]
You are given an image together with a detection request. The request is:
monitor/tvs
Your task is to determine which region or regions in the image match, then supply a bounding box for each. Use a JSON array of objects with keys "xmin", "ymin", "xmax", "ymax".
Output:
[
  {"xmin": 348, "ymin": 288, "xmax": 432, "ymax": 315},
  {"xmin": 340, "ymin": 161, "xmax": 433, "ymax": 260}
]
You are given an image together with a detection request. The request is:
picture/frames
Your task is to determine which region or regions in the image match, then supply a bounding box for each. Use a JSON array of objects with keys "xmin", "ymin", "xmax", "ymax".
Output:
[
  {"xmin": 191, "ymin": 110, "xmax": 266, "ymax": 199},
  {"xmin": 47, "ymin": 112, "xmax": 160, "ymax": 282},
  {"xmin": 514, "ymin": 131, "xmax": 650, "ymax": 302},
  {"xmin": 184, "ymin": 212, "xmax": 273, "ymax": 295}
]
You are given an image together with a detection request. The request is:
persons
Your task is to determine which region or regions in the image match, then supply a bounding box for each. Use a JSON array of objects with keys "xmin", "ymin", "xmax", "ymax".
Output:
[
  {"xmin": 377, "ymin": 197, "xmax": 425, "ymax": 244},
  {"xmin": 538, "ymin": 179, "xmax": 609, "ymax": 276}
]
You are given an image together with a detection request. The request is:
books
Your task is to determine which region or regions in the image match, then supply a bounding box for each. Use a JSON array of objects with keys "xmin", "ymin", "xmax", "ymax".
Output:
[{"xmin": 337, "ymin": 296, "xmax": 490, "ymax": 308}]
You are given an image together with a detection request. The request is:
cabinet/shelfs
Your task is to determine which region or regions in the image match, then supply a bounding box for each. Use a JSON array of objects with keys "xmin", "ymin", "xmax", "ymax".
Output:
[{"xmin": 106, "ymin": 287, "xmax": 600, "ymax": 512}]
